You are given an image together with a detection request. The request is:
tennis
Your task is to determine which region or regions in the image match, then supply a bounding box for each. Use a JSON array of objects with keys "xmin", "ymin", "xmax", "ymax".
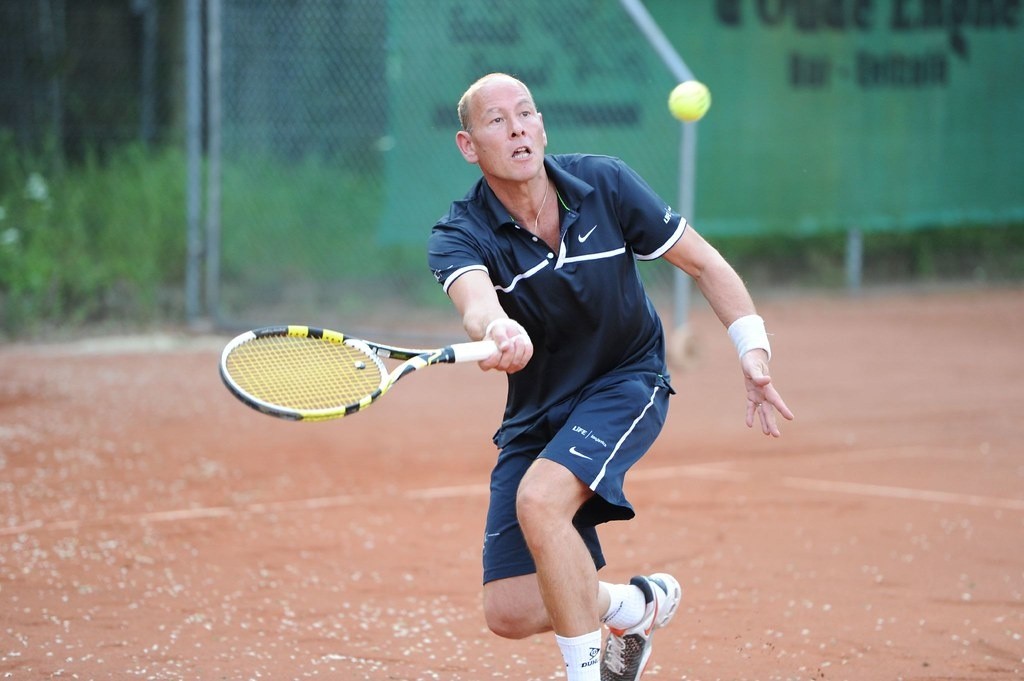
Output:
[{"xmin": 670, "ymin": 79, "xmax": 712, "ymax": 123}]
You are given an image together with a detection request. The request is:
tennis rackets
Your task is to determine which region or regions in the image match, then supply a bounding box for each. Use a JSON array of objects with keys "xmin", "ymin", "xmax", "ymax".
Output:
[{"xmin": 219, "ymin": 327, "xmax": 498, "ymax": 420}]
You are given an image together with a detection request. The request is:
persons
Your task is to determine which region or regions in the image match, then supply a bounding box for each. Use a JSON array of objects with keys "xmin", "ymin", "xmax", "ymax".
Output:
[{"xmin": 429, "ymin": 67, "xmax": 796, "ymax": 680}]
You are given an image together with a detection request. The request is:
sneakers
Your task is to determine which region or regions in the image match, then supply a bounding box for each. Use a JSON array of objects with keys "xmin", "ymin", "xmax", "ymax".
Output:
[{"xmin": 600, "ymin": 572, "xmax": 682, "ymax": 681}]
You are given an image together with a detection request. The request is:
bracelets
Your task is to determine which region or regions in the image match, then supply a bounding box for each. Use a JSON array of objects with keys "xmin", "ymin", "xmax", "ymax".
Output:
[
  {"xmin": 484, "ymin": 318, "xmax": 517, "ymax": 336},
  {"xmin": 728, "ymin": 315, "xmax": 771, "ymax": 361}
]
